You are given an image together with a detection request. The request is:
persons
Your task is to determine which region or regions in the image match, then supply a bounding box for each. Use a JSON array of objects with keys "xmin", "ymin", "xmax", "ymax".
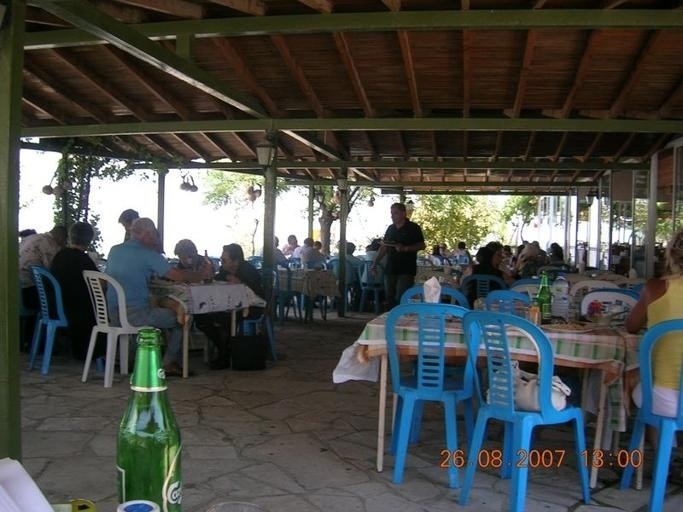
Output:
[
  {"xmin": 18, "ymin": 206, "xmax": 264, "ymax": 374},
  {"xmin": 425, "ymin": 240, "xmax": 564, "ymax": 310},
  {"xmin": 623, "ymin": 222, "xmax": 682, "ymax": 475},
  {"xmin": 274, "ymin": 235, "xmax": 389, "ymax": 312},
  {"xmin": 370, "ymin": 202, "xmax": 426, "ymax": 308}
]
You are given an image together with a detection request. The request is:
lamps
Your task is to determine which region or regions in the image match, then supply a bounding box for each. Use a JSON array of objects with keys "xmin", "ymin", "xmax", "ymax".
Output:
[
  {"xmin": 253, "ymin": 127, "xmax": 282, "ymax": 173},
  {"xmin": 335, "ymin": 171, "xmax": 348, "ymax": 195}
]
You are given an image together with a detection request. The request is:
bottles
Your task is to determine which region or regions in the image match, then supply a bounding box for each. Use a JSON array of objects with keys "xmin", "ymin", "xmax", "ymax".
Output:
[
  {"xmin": 551, "ymin": 270, "xmax": 570, "ymax": 323},
  {"xmin": 537, "ymin": 270, "xmax": 551, "ymax": 322},
  {"xmin": 528, "ymin": 291, "xmax": 541, "ymax": 326},
  {"xmin": 115, "ymin": 326, "xmax": 185, "ymax": 510}
]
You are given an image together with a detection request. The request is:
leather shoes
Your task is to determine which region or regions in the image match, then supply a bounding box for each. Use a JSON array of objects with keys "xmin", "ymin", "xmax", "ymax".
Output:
[{"xmin": 159, "ymin": 361, "xmax": 195, "ymax": 377}]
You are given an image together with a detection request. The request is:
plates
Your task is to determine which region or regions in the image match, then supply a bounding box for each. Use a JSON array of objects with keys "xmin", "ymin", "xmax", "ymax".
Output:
[{"xmin": 540, "ymin": 324, "xmax": 595, "ymax": 334}]
